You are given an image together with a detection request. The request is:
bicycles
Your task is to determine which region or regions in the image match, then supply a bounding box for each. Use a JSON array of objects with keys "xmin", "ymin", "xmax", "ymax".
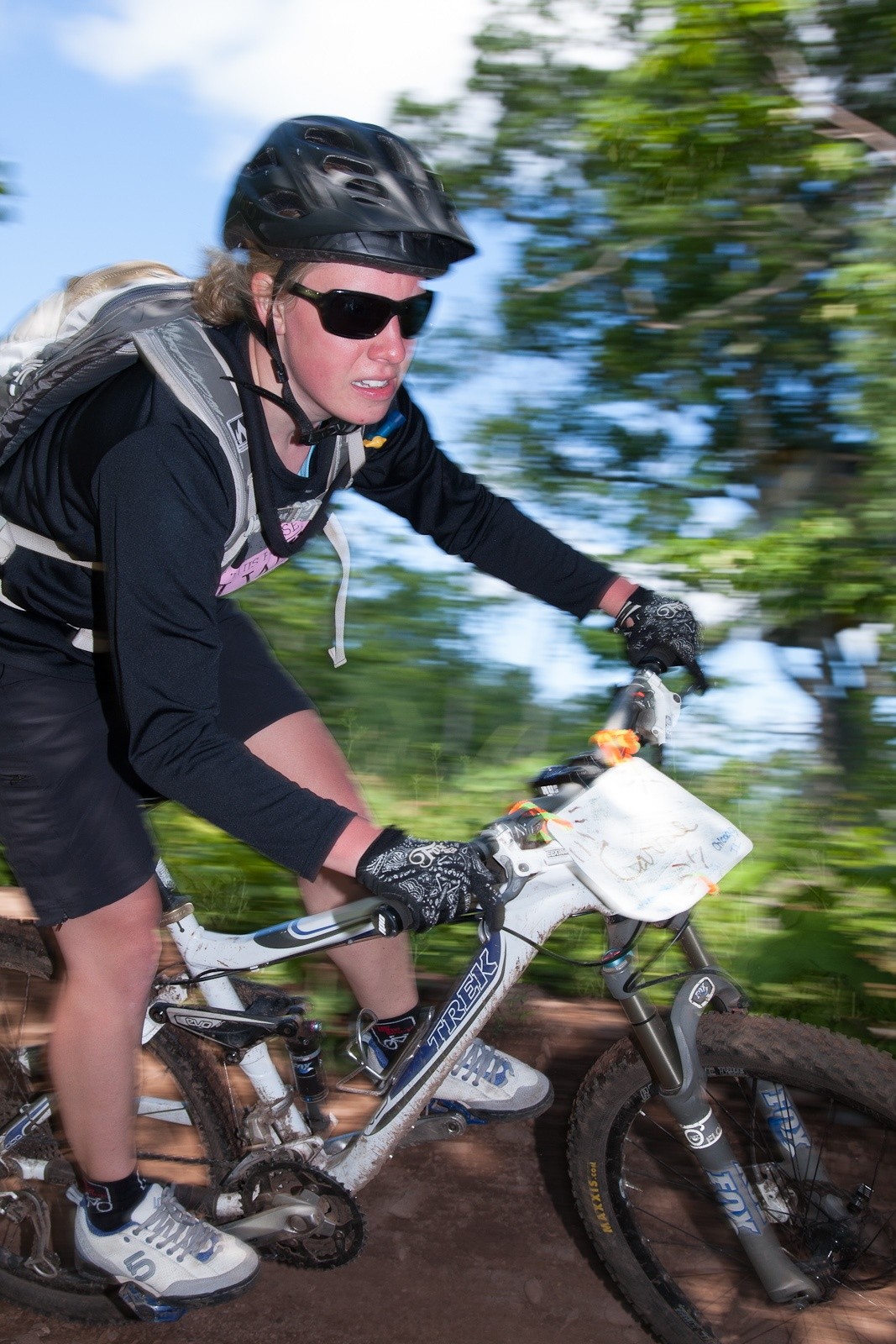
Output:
[{"xmin": 0, "ymin": 654, "xmax": 895, "ymax": 1344}]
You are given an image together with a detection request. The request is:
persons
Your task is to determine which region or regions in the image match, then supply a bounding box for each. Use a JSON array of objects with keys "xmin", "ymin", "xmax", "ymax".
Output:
[{"xmin": 0, "ymin": 115, "xmax": 708, "ymax": 1298}]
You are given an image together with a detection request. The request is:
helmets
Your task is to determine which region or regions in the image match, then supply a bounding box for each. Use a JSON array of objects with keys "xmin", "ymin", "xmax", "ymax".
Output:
[{"xmin": 223, "ymin": 117, "xmax": 476, "ymax": 276}]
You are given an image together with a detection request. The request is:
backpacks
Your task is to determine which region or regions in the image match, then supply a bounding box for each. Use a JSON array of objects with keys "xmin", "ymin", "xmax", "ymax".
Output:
[{"xmin": 0, "ymin": 263, "xmax": 365, "ymax": 668}]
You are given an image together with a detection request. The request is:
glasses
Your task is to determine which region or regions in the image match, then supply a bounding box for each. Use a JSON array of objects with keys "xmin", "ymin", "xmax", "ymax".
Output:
[{"xmin": 272, "ymin": 273, "xmax": 434, "ymax": 338}]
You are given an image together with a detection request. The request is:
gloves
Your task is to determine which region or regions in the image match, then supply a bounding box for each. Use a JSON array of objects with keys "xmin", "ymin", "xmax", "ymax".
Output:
[
  {"xmin": 357, "ymin": 826, "xmax": 510, "ymax": 932},
  {"xmin": 611, "ymin": 587, "xmax": 710, "ymax": 695}
]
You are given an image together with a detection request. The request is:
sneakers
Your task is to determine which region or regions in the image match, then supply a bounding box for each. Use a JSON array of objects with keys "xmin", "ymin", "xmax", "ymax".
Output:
[
  {"xmin": 68, "ymin": 1181, "xmax": 259, "ymax": 1299},
  {"xmin": 357, "ymin": 1011, "xmax": 554, "ymax": 1121}
]
